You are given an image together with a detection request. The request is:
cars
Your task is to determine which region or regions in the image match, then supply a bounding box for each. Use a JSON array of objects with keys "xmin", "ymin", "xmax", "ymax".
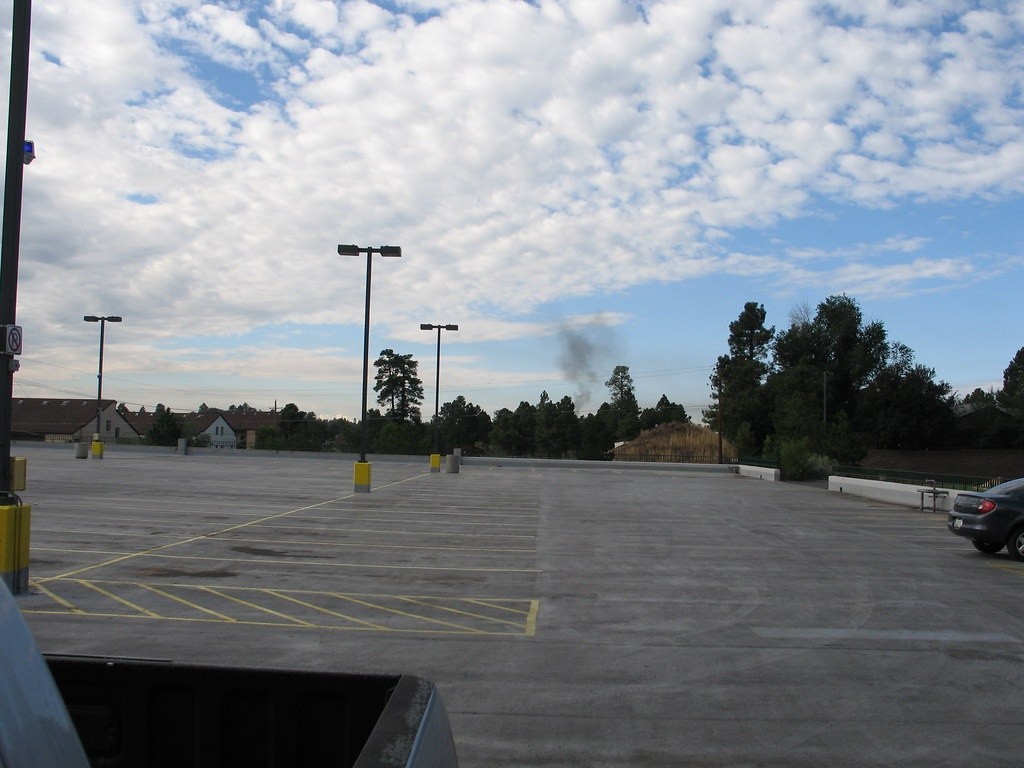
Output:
[{"xmin": 948, "ymin": 478, "xmax": 1024, "ymax": 562}]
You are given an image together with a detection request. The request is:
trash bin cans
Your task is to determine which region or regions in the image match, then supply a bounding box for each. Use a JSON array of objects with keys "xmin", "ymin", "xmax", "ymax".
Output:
[{"xmin": 0, "ymin": 575, "xmax": 461, "ymax": 768}]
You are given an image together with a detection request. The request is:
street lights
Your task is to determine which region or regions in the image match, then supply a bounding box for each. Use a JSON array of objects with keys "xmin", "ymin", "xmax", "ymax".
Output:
[
  {"xmin": 83, "ymin": 315, "xmax": 122, "ymax": 441},
  {"xmin": 338, "ymin": 244, "xmax": 402, "ymax": 463},
  {"xmin": 420, "ymin": 324, "xmax": 458, "ymax": 454}
]
